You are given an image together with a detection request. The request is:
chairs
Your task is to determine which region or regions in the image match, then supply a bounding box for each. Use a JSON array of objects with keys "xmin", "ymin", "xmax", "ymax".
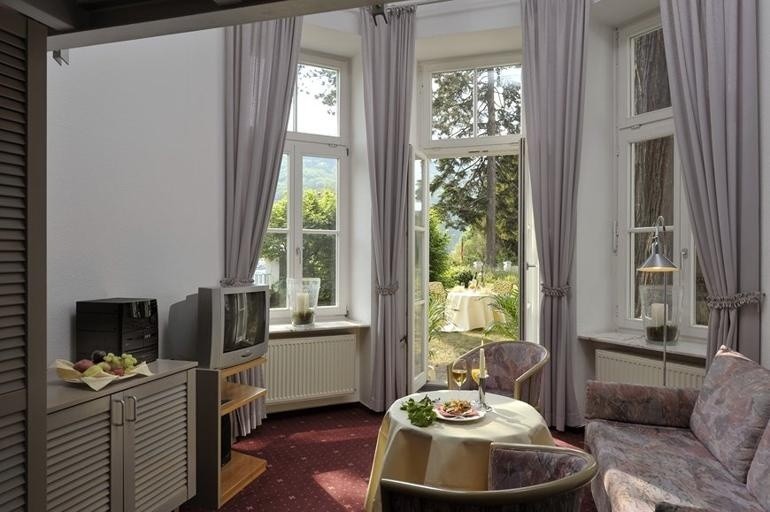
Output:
[
  {"xmin": 379, "ymin": 437, "xmax": 602, "ymax": 511},
  {"xmin": 445, "ymin": 339, "xmax": 551, "ymax": 418},
  {"xmin": 428, "ymin": 279, "xmax": 448, "ymax": 323}
]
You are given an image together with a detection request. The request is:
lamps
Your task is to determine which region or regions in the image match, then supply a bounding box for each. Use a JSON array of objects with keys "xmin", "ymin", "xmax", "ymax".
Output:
[{"xmin": 636, "ymin": 211, "xmax": 684, "ymax": 386}]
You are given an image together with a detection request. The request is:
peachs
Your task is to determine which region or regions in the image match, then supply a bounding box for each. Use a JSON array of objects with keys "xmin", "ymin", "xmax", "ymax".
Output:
[{"xmin": 73, "ymin": 358, "xmax": 95, "ymax": 373}]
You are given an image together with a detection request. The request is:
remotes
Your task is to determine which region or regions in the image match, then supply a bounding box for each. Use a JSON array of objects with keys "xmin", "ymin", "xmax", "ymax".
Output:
[{"xmin": 217, "ymin": 276, "xmax": 255, "ymax": 286}]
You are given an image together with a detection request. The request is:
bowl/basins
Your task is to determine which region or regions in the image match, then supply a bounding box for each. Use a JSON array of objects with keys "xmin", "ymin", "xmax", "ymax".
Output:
[{"xmin": 218, "ymin": 276, "xmax": 235, "ymax": 288}]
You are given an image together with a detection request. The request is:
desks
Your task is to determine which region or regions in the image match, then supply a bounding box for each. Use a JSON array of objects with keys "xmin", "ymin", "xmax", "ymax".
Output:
[{"xmin": 436, "ymin": 288, "xmax": 509, "ymax": 335}]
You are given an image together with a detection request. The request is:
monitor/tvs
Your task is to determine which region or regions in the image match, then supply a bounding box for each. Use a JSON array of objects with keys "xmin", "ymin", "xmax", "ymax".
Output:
[{"xmin": 169, "ymin": 284, "xmax": 269, "ymax": 369}]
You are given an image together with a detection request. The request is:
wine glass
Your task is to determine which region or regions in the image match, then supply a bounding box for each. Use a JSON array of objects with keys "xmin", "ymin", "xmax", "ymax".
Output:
[
  {"xmin": 451, "ymin": 357, "xmax": 468, "ymax": 400},
  {"xmin": 471, "ymin": 357, "xmax": 489, "ymax": 405}
]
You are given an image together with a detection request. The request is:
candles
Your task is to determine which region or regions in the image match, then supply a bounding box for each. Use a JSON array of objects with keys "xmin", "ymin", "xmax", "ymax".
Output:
[
  {"xmin": 650, "ymin": 299, "xmax": 670, "ymax": 330},
  {"xmin": 296, "ymin": 291, "xmax": 310, "ymax": 316},
  {"xmin": 478, "ymin": 348, "xmax": 486, "ymax": 378}
]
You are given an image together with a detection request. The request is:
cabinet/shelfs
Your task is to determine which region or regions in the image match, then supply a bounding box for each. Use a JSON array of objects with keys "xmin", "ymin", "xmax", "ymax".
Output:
[
  {"xmin": 191, "ymin": 355, "xmax": 270, "ymax": 512},
  {"xmin": 44, "ymin": 356, "xmax": 202, "ymax": 512}
]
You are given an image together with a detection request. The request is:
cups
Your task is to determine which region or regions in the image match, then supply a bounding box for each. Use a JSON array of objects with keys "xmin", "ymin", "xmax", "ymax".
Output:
[
  {"xmin": 287, "ymin": 276, "xmax": 321, "ymax": 327},
  {"xmin": 638, "ymin": 284, "xmax": 685, "ymax": 345}
]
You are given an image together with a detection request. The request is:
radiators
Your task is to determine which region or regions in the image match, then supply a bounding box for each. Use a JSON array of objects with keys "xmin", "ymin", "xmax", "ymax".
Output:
[
  {"xmin": 260, "ymin": 331, "xmax": 357, "ymax": 409},
  {"xmin": 592, "ymin": 343, "xmax": 709, "ymax": 392}
]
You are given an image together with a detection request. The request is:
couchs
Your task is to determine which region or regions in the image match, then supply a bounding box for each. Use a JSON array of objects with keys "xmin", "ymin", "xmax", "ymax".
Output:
[{"xmin": 578, "ymin": 344, "xmax": 769, "ymax": 512}]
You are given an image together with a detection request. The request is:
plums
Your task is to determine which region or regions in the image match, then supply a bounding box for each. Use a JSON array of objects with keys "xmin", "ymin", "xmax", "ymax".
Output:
[{"xmin": 91, "ymin": 350, "xmax": 106, "ymax": 364}]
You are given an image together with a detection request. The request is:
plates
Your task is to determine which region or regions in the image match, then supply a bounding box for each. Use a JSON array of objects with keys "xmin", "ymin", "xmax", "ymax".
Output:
[
  {"xmin": 64, "ymin": 373, "xmax": 137, "ymax": 385},
  {"xmin": 237, "ymin": 278, "xmax": 255, "ymax": 286},
  {"xmin": 432, "ymin": 404, "xmax": 487, "ymax": 423}
]
